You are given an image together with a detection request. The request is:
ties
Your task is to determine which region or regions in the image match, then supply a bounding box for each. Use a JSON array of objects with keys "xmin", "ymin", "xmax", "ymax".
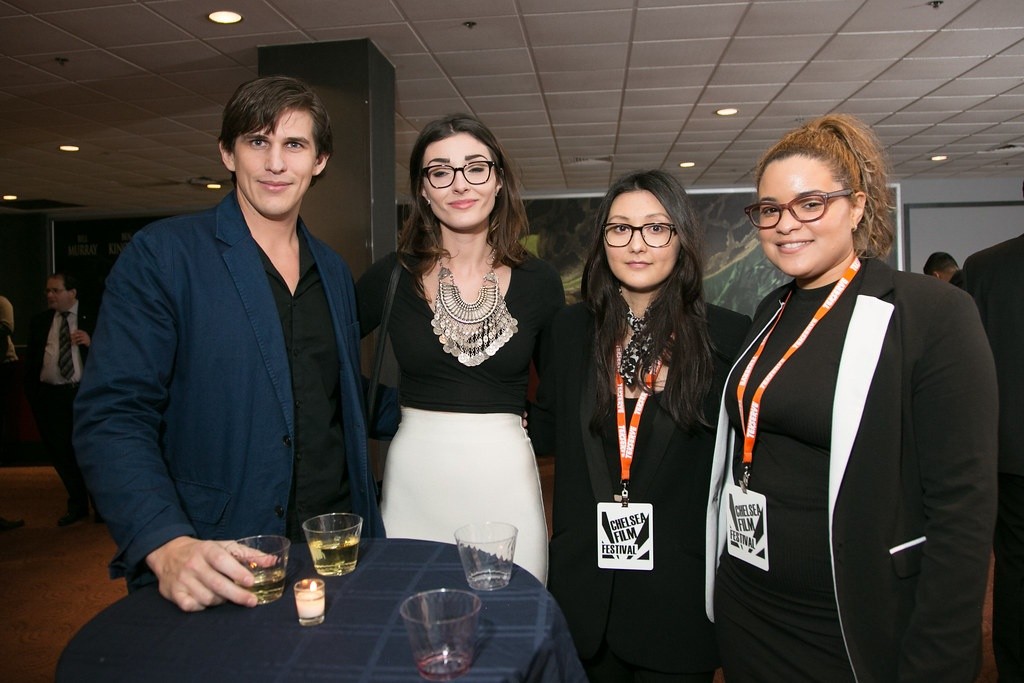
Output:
[{"xmin": 58, "ymin": 312, "xmax": 75, "ymax": 380}]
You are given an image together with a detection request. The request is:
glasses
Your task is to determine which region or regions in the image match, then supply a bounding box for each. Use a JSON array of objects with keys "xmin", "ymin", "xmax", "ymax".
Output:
[
  {"xmin": 422, "ymin": 161, "xmax": 499, "ymax": 188},
  {"xmin": 46, "ymin": 288, "xmax": 67, "ymax": 294},
  {"xmin": 602, "ymin": 222, "xmax": 676, "ymax": 248},
  {"xmin": 744, "ymin": 190, "xmax": 853, "ymax": 229}
]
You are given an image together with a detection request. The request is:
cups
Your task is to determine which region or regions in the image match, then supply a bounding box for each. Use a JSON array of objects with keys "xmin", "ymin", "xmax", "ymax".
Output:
[
  {"xmin": 302, "ymin": 513, "xmax": 363, "ymax": 576},
  {"xmin": 399, "ymin": 588, "xmax": 482, "ymax": 680},
  {"xmin": 454, "ymin": 522, "xmax": 518, "ymax": 591},
  {"xmin": 69, "ymin": 329, "xmax": 76, "ymax": 346},
  {"xmin": 225, "ymin": 535, "xmax": 291, "ymax": 604}
]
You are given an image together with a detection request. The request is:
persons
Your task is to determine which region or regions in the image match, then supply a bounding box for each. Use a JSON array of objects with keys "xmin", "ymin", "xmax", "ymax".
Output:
[
  {"xmin": 71, "ymin": 76, "xmax": 382, "ymax": 612},
  {"xmin": 704, "ymin": 112, "xmax": 999, "ymax": 683},
  {"xmin": 923, "ymin": 251, "xmax": 962, "ymax": 289},
  {"xmin": 352, "ymin": 113, "xmax": 565, "ymax": 586},
  {"xmin": 549, "ymin": 168, "xmax": 750, "ymax": 683},
  {"xmin": 962, "ymin": 179, "xmax": 1024, "ymax": 683},
  {"xmin": 0, "ymin": 295, "xmax": 25, "ymax": 465},
  {"xmin": 25, "ymin": 272, "xmax": 105, "ymax": 526}
]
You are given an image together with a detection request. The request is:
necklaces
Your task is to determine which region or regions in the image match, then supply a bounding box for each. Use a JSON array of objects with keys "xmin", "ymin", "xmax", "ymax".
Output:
[{"xmin": 430, "ymin": 249, "xmax": 519, "ymax": 366}]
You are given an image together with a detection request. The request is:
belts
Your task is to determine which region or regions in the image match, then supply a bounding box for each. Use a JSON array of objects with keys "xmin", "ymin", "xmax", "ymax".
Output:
[{"xmin": 37, "ymin": 382, "xmax": 82, "ymax": 390}]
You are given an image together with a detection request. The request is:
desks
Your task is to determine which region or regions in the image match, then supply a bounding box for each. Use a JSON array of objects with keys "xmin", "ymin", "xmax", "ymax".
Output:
[{"xmin": 53, "ymin": 539, "xmax": 587, "ymax": 683}]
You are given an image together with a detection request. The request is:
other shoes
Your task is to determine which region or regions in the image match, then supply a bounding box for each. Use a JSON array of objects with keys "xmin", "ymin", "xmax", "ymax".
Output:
[
  {"xmin": 94, "ymin": 511, "xmax": 104, "ymax": 523},
  {"xmin": 0, "ymin": 517, "xmax": 25, "ymax": 532},
  {"xmin": 57, "ymin": 510, "xmax": 89, "ymax": 526}
]
